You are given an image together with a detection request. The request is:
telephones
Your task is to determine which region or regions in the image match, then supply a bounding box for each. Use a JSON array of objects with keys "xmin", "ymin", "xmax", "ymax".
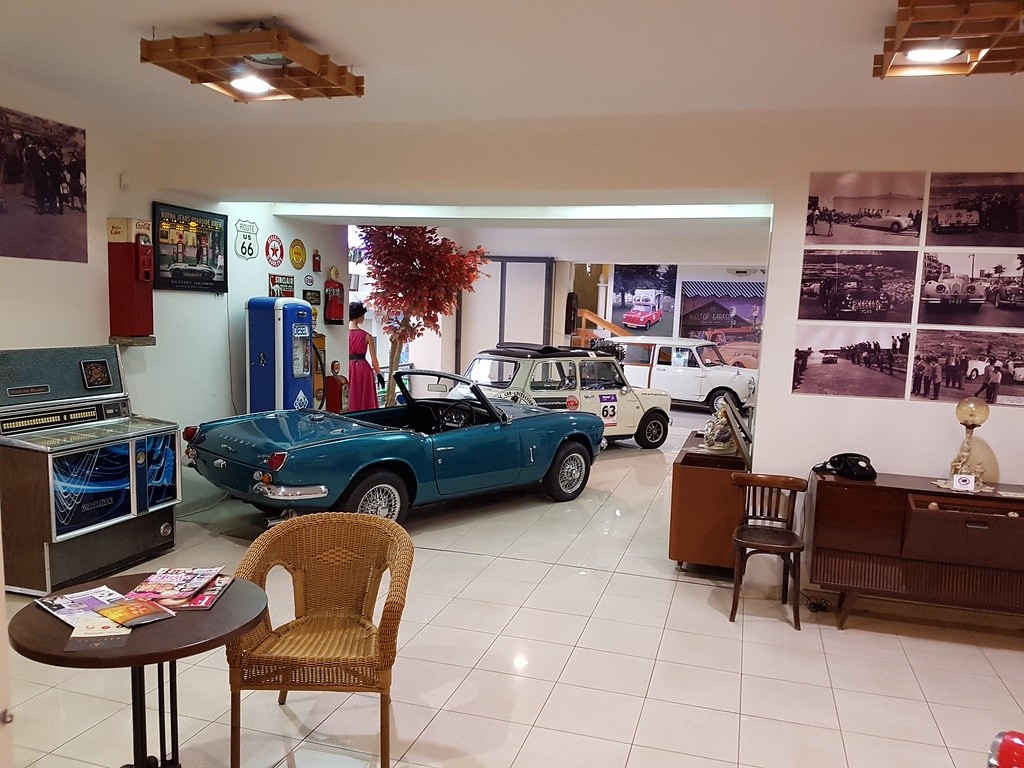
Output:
[{"xmin": 830, "ymin": 453, "xmax": 877, "ymax": 480}]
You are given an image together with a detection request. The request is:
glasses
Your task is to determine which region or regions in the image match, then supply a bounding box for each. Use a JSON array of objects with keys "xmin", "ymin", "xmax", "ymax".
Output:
[{"xmin": 206, "ymin": 589, "xmax": 216, "ymax": 591}]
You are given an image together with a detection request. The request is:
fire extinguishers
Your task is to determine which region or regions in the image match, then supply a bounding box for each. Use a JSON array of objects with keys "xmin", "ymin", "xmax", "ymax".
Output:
[{"xmin": 323, "ymin": 266, "xmax": 344, "ymax": 325}]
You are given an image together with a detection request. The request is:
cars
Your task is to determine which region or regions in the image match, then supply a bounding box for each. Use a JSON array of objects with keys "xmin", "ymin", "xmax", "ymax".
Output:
[
  {"xmin": 987, "ymin": 280, "xmax": 1023, "ymax": 309},
  {"xmin": 623, "ymin": 304, "xmax": 664, "ymax": 330},
  {"xmin": 976, "ymin": 277, "xmax": 990, "ymax": 290},
  {"xmin": 445, "ymin": 342, "xmax": 674, "ymax": 450},
  {"xmin": 181, "ymin": 370, "xmax": 605, "ymax": 528},
  {"xmin": 690, "ymin": 328, "xmax": 760, "ymax": 371},
  {"xmin": 822, "ymin": 354, "xmax": 838, "ymax": 364},
  {"xmin": 941, "ymin": 344, "xmax": 968, "ymax": 356},
  {"xmin": 823, "ymin": 281, "xmax": 891, "ymax": 320},
  {"xmin": 605, "ymin": 336, "xmax": 757, "ymax": 415},
  {"xmin": 966, "ymin": 356, "xmax": 1003, "ymax": 381},
  {"xmin": 920, "ymin": 273, "xmax": 986, "ymax": 312},
  {"xmin": 850, "ymin": 212, "xmax": 913, "ymax": 232},
  {"xmin": 1003, "ymin": 360, "xmax": 1024, "ymax": 384},
  {"xmin": 931, "ymin": 204, "xmax": 980, "ymax": 235}
]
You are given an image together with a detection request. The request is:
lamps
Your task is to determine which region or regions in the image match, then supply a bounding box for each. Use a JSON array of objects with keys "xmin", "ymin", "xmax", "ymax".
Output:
[
  {"xmin": 872, "ymin": 0, "xmax": 1024, "ymax": 76},
  {"xmin": 950, "ymin": 397, "xmax": 990, "ymax": 475},
  {"xmin": 140, "ymin": 17, "xmax": 364, "ymax": 104}
]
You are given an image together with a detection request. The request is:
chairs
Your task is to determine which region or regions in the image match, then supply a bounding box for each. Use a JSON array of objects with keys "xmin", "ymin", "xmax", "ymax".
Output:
[
  {"xmin": 729, "ymin": 473, "xmax": 808, "ymax": 630},
  {"xmin": 225, "ymin": 512, "xmax": 414, "ymax": 768}
]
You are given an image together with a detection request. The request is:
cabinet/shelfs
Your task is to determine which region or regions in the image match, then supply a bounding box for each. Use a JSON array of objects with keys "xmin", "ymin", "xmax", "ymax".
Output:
[
  {"xmin": 668, "ymin": 393, "xmax": 753, "ymax": 572},
  {"xmin": 808, "ymin": 470, "xmax": 1024, "ymax": 630}
]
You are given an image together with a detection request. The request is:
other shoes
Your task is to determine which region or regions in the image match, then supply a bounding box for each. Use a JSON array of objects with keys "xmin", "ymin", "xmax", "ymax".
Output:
[
  {"xmin": 975, "ymin": 393, "xmax": 996, "ymax": 404},
  {"xmin": 911, "ymin": 391, "xmax": 938, "ymax": 400}
]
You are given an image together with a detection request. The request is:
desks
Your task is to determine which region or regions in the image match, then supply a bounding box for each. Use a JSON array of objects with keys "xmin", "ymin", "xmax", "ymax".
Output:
[{"xmin": 8, "ymin": 572, "xmax": 268, "ymax": 768}]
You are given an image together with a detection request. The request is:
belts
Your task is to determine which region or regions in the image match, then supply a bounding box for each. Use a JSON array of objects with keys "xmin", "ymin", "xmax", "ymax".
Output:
[{"xmin": 947, "ymin": 365, "xmax": 956, "ymax": 368}]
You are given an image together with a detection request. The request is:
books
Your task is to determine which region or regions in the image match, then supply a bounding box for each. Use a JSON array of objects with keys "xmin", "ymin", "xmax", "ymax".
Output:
[{"xmin": 124, "ymin": 564, "xmax": 236, "ymax": 610}]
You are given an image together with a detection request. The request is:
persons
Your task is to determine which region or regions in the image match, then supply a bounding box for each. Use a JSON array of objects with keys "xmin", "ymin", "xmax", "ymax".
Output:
[
  {"xmin": 953, "ymin": 192, "xmax": 1024, "ymax": 236},
  {"xmin": 1006, "ymin": 277, "xmax": 1017, "ymax": 286},
  {"xmin": 974, "ymin": 359, "xmax": 1002, "ymax": 404},
  {"xmin": 857, "ymin": 208, "xmax": 890, "ymax": 223},
  {"xmin": 806, "ymin": 205, "xmax": 844, "ymax": 237},
  {"xmin": 349, "ymin": 301, "xmax": 385, "ymax": 412},
  {"xmin": 840, "ymin": 340, "xmax": 895, "ymax": 375},
  {"xmin": 1, "ymin": 127, "xmax": 86, "ymax": 215},
  {"xmin": 911, "ymin": 355, "xmax": 942, "ymax": 400},
  {"xmin": 987, "ymin": 342, "xmax": 993, "ymax": 354},
  {"xmin": 946, "ymin": 352, "xmax": 968, "ymax": 390},
  {"xmin": 870, "ymin": 273, "xmax": 883, "ymax": 291},
  {"xmin": 1008, "ymin": 351, "xmax": 1017, "ymax": 356},
  {"xmin": 892, "ymin": 336, "xmax": 897, "ymax": 354},
  {"xmin": 908, "ymin": 210, "xmax": 922, "ymax": 237},
  {"xmin": 897, "ymin": 332, "xmax": 910, "ymax": 354},
  {"xmin": 996, "ymin": 277, "xmax": 1000, "ymax": 284}
]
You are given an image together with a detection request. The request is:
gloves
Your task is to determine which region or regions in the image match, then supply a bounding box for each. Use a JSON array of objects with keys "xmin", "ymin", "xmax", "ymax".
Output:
[{"xmin": 377, "ymin": 374, "xmax": 385, "ymax": 389}]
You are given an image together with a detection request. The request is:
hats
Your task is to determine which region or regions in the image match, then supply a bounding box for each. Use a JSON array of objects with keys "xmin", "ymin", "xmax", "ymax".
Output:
[
  {"xmin": 348, "ymin": 302, "xmax": 366, "ymax": 319},
  {"xmin": 995, "ymin": 366, "xmax": 1001, "ymax": 371}
]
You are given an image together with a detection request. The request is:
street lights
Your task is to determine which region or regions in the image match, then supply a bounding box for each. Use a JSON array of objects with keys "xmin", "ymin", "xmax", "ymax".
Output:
[{"xmin": 968, "ymin": 253, "xmax": 974, "ymax": 278}]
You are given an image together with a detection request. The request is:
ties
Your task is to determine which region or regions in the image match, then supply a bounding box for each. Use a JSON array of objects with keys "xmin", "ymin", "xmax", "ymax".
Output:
[{"xmin": 951, "ymin": 357, "xmax": 954, "ymax": 363}]
